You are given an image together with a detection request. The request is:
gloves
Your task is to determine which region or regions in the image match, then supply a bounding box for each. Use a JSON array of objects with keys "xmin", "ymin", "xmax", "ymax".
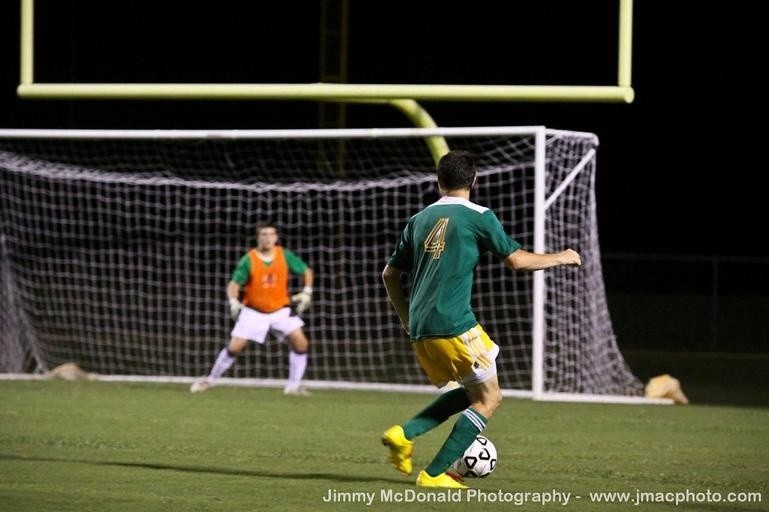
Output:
[
  {"xmin": 230, "ymin": 298, "xmax": 244, "ymax": 321},
  {"xmin": 291, "ymin": 287, "xmax": 313, "ymax": 315}
]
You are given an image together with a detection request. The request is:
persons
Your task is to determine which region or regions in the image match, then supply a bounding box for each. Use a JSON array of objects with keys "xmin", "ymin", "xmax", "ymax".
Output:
[
  {"xmin": 189, "ymin": 224, "xmax": 318, "ymax": 398},
  {"xmin": 378, "ymin": 147, "xmax": 583, "ymax": 490}
]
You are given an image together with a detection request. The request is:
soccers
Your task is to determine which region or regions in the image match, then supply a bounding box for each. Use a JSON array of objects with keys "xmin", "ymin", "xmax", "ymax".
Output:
[{"xmin": 452, "ymin": 432, "xmax": 497, "ymax": 479}]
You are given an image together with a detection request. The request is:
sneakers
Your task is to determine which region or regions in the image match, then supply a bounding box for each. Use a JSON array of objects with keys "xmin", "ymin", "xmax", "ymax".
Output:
[
  {"xmin": 416, "ymin": 469, "xmax": 470, "ymax": 489},
  {"xmin": 191, "ymin": 375, "xmax": 217, "ymax": 393},
  {"xmin": 282, "ymin": 385, "xmax": 308, "ymax": 396},
  {"xmin": 382, "ymin": 424, "xmax": 414, "ymax": 476}
]
